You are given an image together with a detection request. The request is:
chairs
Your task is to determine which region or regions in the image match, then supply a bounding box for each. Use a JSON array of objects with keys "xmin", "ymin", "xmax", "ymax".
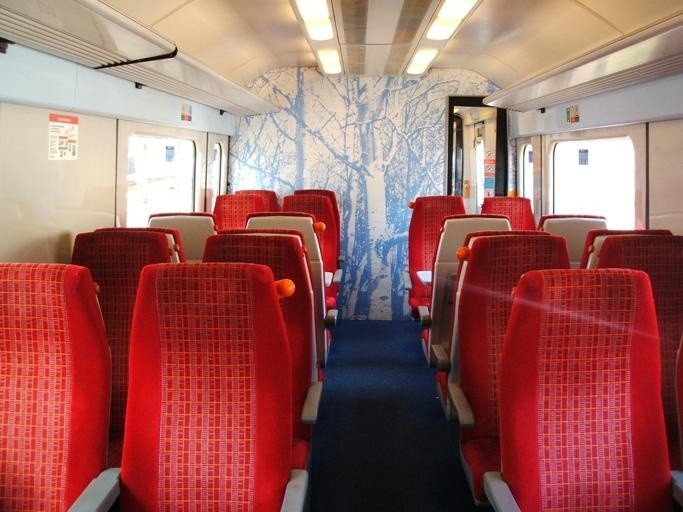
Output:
[
  {"xmin": 406, "ymin": 194, "xmax": 682, "ymax": 511},
  {"xmin": 0, "ymin": 188, "xmax": 341, "ymax": 512}
]
